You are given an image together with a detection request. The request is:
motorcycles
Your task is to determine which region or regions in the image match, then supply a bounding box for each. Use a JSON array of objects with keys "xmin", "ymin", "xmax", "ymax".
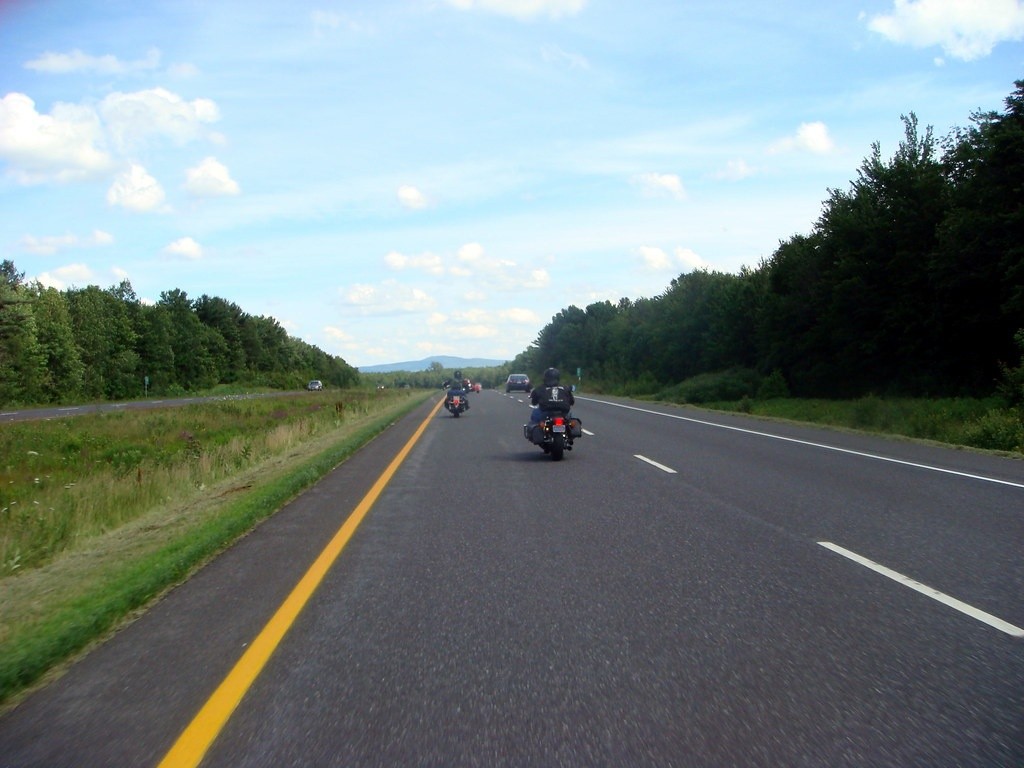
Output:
[
  {"xmin": 524, "ymin": 393, "xmax": 582, "ymax": 460},
  {"xmin": 444, "ymin": 381, "xmax": 471, "ymax": 418}
]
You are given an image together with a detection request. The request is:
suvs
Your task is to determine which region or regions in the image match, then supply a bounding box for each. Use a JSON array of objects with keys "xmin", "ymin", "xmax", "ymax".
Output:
[{"xmin": 505, "ymin": 373, "xmax": 532, "ymax": 394}]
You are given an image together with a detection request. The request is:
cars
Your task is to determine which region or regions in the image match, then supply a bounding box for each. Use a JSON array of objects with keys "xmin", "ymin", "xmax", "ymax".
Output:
[{"xmin": 308, "ymin": 381, "xmax": 322, "ymax": 391}]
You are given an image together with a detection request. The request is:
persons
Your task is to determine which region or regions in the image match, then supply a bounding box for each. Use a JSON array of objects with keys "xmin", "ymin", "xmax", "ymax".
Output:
[
  {"xmin": 531, "ymin": 367, "xmax": 574, "ymax": 448},
  {"xmin": 444, "ymin": 370, "xmax": 471, "ymax": 410}
]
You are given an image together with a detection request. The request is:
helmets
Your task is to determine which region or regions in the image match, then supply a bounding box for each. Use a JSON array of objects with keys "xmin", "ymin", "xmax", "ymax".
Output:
[
  {"xmin": 544, "ymin": 368, "xmax": 560, "ymax": 383},
  {"xmin": 454, "ymin": 370, "xmax": 461, "ymax": 378}
]
